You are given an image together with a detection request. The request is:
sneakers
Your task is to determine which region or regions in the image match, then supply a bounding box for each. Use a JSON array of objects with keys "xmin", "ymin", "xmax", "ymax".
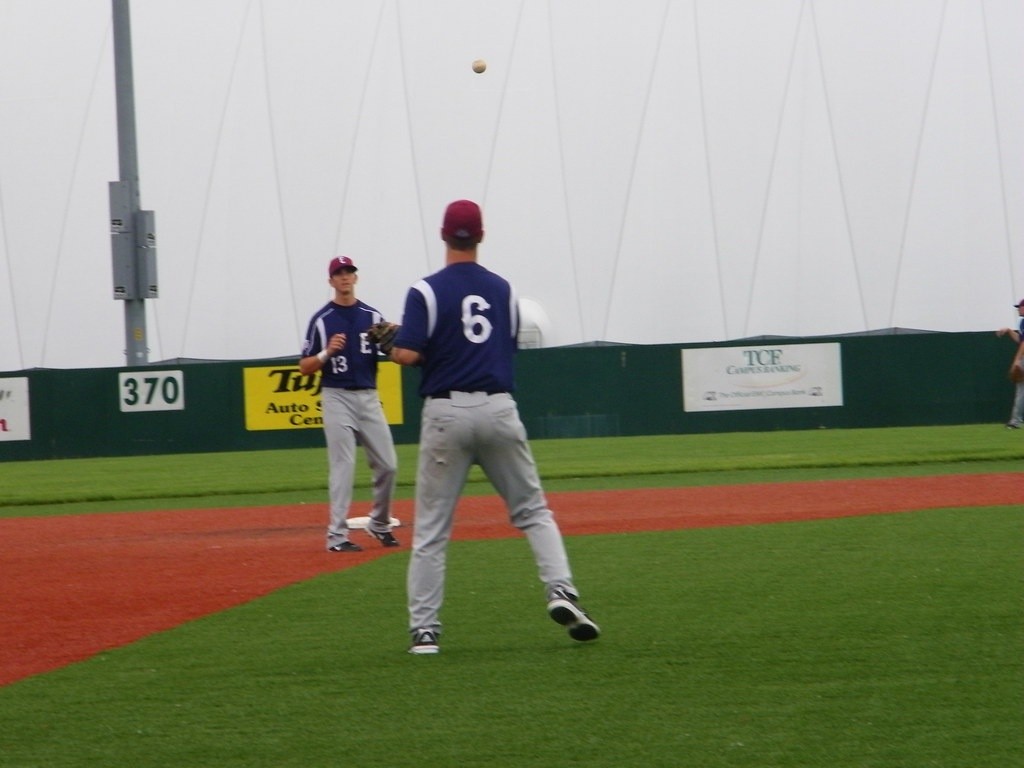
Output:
[
  {"xmin": 366, "ymin": 527, "xmax": 399, "ymax": 546},
  {"xmin": 547, "ymin": 586, "xmax": 601, "ymax": 642},
  {"xmin": 409, "ymin": 628, "xmax": 440, "ymax": 655},
  {"xmin": 328, "ymin": 542, "xmax": 360, "ymax": 553}
]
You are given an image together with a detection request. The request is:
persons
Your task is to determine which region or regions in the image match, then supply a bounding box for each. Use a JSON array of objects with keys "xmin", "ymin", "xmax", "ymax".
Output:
[
  {"xmin": 381, "ymin": 200, "xmax": 602, "ymax": 655},
  {"xmin": 299, "ymin": 255, "xmax": 400, "ymax": 552},
  {"xmin": 995, "ymin": 299, "xmax": 1024, "ymax": 428}
]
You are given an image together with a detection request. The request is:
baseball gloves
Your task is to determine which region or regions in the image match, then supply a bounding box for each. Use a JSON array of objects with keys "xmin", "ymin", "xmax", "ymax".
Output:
[{"xmin": 368, "ymin": 321, "xmax": 402, "ymax": 356}]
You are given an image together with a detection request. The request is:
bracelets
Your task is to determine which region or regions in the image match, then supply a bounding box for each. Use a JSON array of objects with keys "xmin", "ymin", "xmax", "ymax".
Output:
[{"xmin": 315, "ymin": 348, "xmax": 330, "ymax": 363}]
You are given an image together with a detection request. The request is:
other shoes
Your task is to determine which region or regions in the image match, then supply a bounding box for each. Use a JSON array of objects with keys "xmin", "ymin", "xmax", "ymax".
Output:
[{"xmin": 1006, "ymin": 423, "xmax": 1018, "ymax": 430}]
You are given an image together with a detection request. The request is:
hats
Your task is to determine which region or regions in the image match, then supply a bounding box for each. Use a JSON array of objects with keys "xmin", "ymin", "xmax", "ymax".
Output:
[
  {"xmin": 444, "ymin": 200, "xmax": 482, "ymax": 239},
  {"xmin": 1014, "ymin": 299, "xmax": 1024, "ymax": 307},
  {"xmin": 328, "ymin": 256, "xmax": 358, "ymax": 275}
]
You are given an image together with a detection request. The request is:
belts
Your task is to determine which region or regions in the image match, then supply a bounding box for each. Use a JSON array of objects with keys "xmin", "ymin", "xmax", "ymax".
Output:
[{"xmin": 427, "ymin": 388, "xmax": 506, "ymax": 399}]
